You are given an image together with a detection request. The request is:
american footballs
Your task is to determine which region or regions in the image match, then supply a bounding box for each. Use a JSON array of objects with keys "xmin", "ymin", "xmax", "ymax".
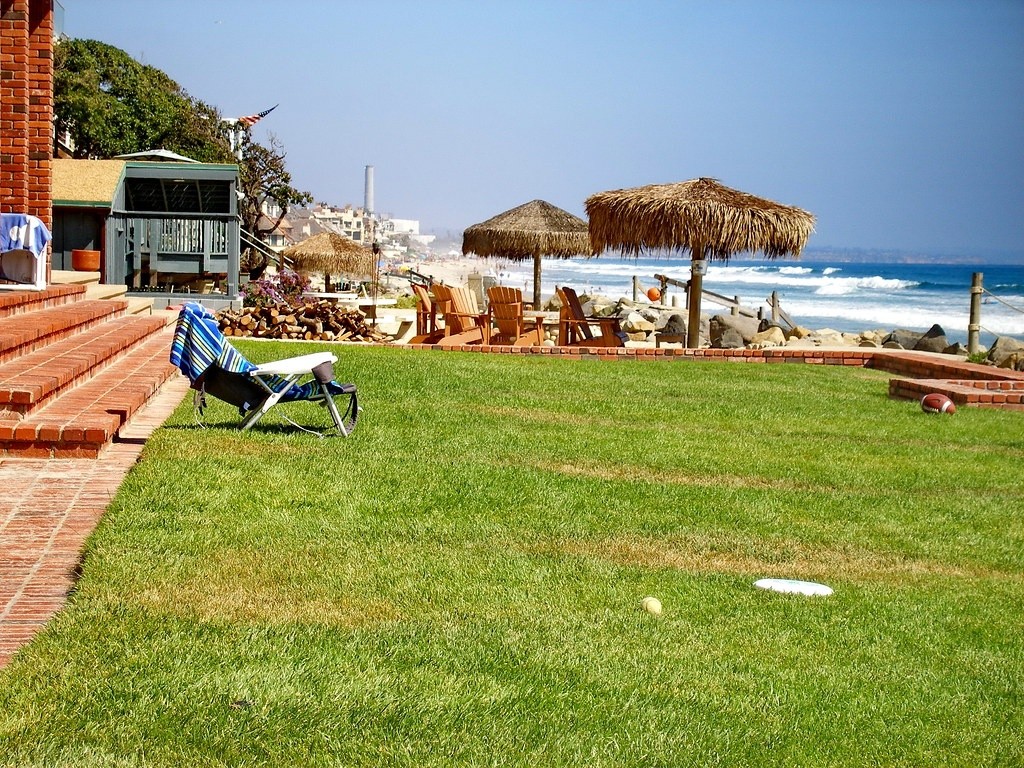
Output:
[{"xmin": 919, "ymin": 392, "xmax": 956, "ymax": 415}]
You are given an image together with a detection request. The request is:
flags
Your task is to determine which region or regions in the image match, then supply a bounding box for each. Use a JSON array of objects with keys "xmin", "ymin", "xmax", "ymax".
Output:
[{"xmin": 240, "ymin": 106, "xmax": 277, "ymax": 127}]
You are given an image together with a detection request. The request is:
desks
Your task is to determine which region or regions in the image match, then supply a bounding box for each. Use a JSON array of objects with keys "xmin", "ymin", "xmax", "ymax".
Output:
[
  {"xmin": 302, "ymin": 291, "xmax": 398, "ymax": 328},
  {"xmin": 520, "ymin": 311, "xmax": 561, "ymax": 342},
  {"xmin": 656, "ymin": 331, "xmax": 686, "ymax": 348}
]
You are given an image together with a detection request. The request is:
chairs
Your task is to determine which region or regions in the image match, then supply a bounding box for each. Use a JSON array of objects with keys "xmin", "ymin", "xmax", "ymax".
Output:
[
  {"xmin": 407, "ymin": 283, "xmax": 496, "ymax": 345},
  {"xmin": 555, "ymin": 285, "xmax": 629, "ymax": 348},
  {"xmin": 170, "ymin": 303, "xmax": 363, "ymax": 440},
  {"xmin": 0, "ymin": 212, "xmax": 53, "ymax": 292},
  {"xmin": 482, "ymin": 286, "xmax": 546, "ymax": 346}
]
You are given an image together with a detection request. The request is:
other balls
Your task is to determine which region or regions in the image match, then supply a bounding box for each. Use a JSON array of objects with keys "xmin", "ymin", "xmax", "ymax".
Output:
[{"xmin": 647, "ymin": 287, "xmax": 661, "ymax": 301}]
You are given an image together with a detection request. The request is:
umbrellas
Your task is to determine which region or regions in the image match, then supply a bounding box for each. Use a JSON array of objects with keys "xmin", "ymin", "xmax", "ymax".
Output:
[
  {"xmin": 283, "ymin": 231, "xmax": 376, "ymax": 292},
  {"xmin": 588, "ymin": 177, "xmax": 816, "ymax": 350},
  {"xmin": 462, "ymin": 200, "xmax": 594, "ymax": 310}
]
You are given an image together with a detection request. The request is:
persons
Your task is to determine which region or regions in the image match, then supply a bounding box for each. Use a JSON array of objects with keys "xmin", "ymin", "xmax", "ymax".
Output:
[
  {"xmin": 473, "ymin": 258, "xmax": 510, "ymax": 285},
  {"xmin": 388, "ymin": 260, "xmax": 391, "ymax": 268},
  {"xmin": 529, "ymin": 281, "xmax": 530, "ymax": 291},
  {"xmin": 416, "ymin": 263, "xmax": 419, "ymax": 272},
  {"xmin": 590, "ymin": 286, "xmax": 593, "ymax": 295}
]
